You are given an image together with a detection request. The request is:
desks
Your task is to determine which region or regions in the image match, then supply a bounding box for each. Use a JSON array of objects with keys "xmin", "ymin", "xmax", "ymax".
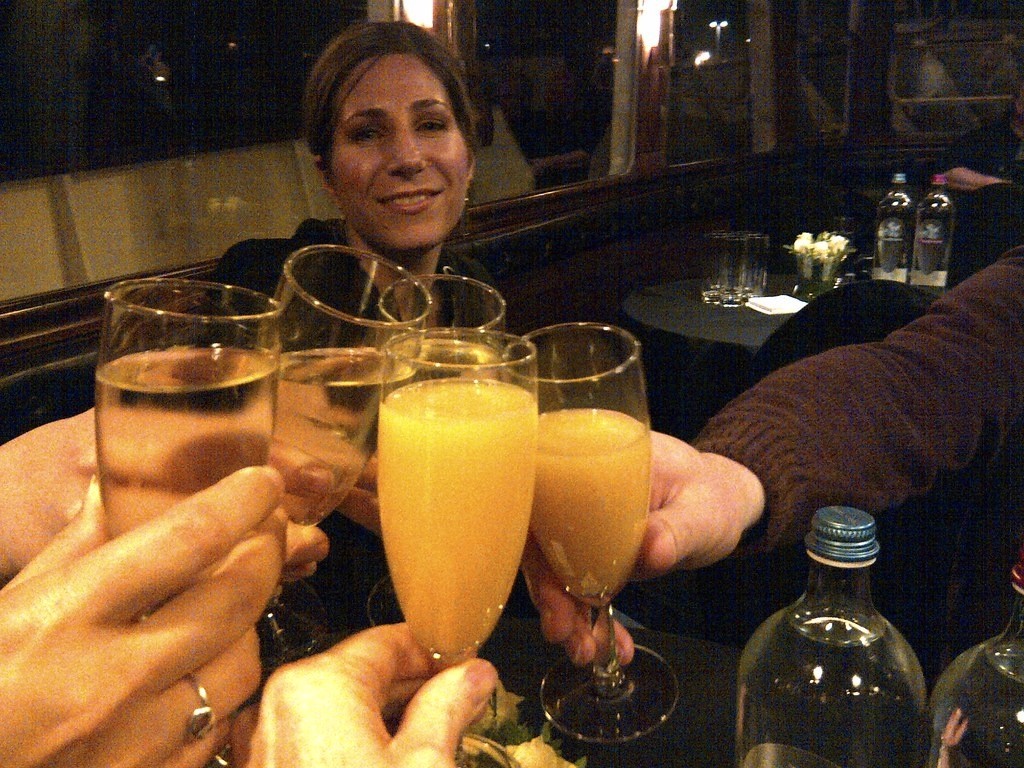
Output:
[
  {"xmin": 264, "ymin": 615, "xmax": 739, "ymax": 768},
  {"xmin": 625, "ymin": 271, "xmax": 861, "ymax": 367}
]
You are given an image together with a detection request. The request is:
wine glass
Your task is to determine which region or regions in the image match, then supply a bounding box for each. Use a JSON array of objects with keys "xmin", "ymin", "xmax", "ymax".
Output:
[{"xmin": 95, "ymin": 244, "xmax": 679, "ymax": 768}]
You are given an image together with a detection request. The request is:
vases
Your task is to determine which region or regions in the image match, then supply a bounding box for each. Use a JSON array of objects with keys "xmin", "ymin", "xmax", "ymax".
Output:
[{"xmin": 792, "ymin": 257, "xmax": 847, "ymax": 297}]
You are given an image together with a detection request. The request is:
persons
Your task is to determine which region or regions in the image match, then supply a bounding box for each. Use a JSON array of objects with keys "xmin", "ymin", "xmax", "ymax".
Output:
[
  {"xmin": 0, "ymin": 405, "xmax": 499, "ymax": 768},
  {"xmin": 211, "ymin": 21, "xmax": 492, "ymax": 638},
  {"xmin": 938, "ymin": 82, "xmax": 1024, "ymax": 192},
  {"xmin": 521, "ymin": 242, "xmax": 1024, "ymax": 669},
  {"xmin": 511, "ymin": 45, "xmax": 603, "ymax": 186}
]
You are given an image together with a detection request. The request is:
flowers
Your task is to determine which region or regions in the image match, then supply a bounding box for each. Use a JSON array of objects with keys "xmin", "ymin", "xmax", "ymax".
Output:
[{"xmin": 788, "ymin": 231, "xmax": 856, "ymax": 283}]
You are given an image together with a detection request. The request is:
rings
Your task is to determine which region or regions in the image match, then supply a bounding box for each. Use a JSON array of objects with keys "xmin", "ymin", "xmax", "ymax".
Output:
[{"xmin": 183, "ymin": 671, "xmax": 219, "ymax": 746}]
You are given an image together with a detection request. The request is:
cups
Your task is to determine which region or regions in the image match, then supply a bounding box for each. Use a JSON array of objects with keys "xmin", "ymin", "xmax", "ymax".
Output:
[{"xmin": 700, "ymin": 231, "xmax": 769, "ymax": 308}]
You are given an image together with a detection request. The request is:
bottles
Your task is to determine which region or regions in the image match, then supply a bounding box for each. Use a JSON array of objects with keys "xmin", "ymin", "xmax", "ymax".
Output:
[
  {"xmin": 734, "ymin": 506, "xmax": 930, "ymax": 768},
  {"xmin": 872, "ymin": 173, "xmax": 914, "ymax": 283},
  {"xmin": 911, "ymin": 175, "xmax": 954, "ymax": 285},
  {"xmin": 926, "ymin": 549, "xmax": 1024, "ymax": 768}
]
